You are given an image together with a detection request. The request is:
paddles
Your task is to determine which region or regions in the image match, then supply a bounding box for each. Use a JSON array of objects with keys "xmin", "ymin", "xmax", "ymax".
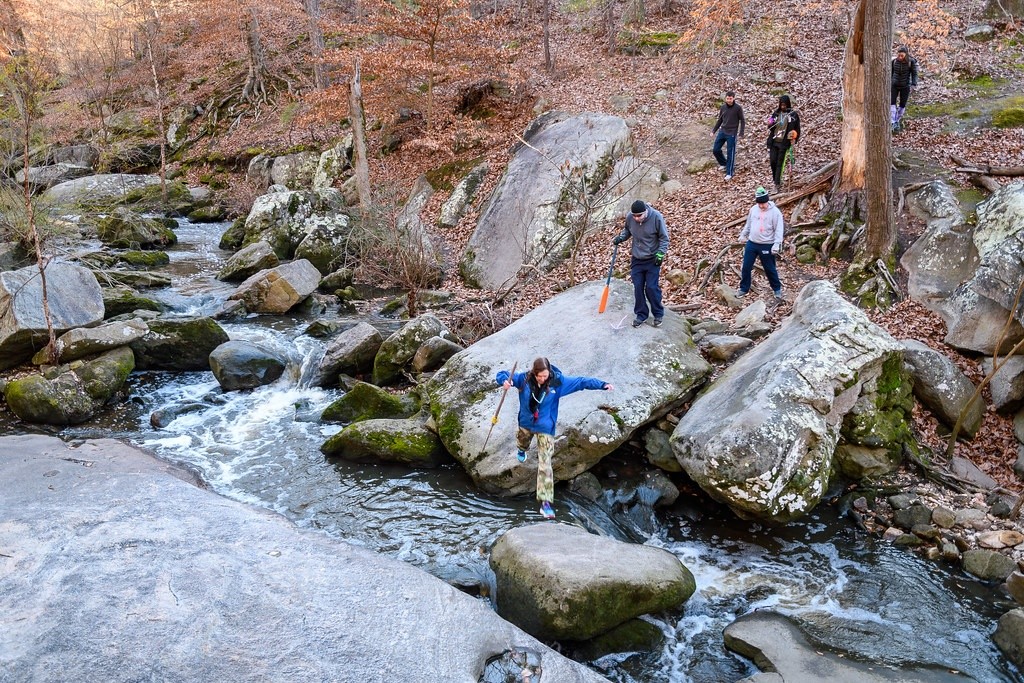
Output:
[
  {"xmin": 481, "ymin": 361, "xmax": 518, "ymax": 452},
  {"xmin": 598, "ymin": 227, "xmax": 622, "ymax": 314}
]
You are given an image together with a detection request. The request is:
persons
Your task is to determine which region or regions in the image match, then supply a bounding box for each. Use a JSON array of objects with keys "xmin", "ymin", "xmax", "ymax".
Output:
[
  {"xmin": 709, "ymin": 91, "xmax": 745, "ymax": 181},
  {"xmin": 734, "ymin": 185, "xmax": 784, "ymax": 300},
  {"xmin": 496, "ymin": 357, "xmax": 614, "ymax": 519},
  {"xmin": 767, "ymin": 94, "xmax": 801, "ymax": 196},
  {"xmin": 891, "ymin": 47, "xmax": 918, "ymax": 131},
  {"xmin": 613, "ymin": 199, "xmax": 670, "ymax": 329}
]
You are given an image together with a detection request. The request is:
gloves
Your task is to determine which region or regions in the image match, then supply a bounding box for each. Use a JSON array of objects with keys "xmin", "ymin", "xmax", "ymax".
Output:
[
  {"xmin": 613, "ymin": 236, "xmax": 623, "ymax": 245},
  {"xmin": 655, "ymin": 250, "xmax": 663, "ymax": 266}
]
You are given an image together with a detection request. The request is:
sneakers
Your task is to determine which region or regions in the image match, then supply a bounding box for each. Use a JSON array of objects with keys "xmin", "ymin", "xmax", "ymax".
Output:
[
  {"xmin": 771, "ymin": 179, "xmax": 779, "ymax": 195},
  {"xmin": 517, "ymin": 447, "xmax": 527, "ymax": 464},
  {"xmin": 734, "ymin": 290, "xmax": 750, "ymax": 299},
  {"xmin": 718, "ymin": 164, "xmax": 733, "ymax": 181},
  {"xmin": 539, "ymin": 500, "xmax": 555, "ymax": 520},
  {"xmin": 774, "ymin": 289, "xmax": 782, "ymax": 299},
  {"xmin": 632, "ymin": 316, "xmax": 663, "ymax": 328}
]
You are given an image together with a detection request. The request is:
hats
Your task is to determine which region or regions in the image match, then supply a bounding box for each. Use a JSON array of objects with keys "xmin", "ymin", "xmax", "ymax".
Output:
[
  {"xmin": 755, "ymin": 187, "xmax": 769, "ymax": 203},
  {"xmin": 779, "ymin": 95, "xmax": 791, "ymax": 113},
  {"xmin": 631, "ymin": 200, "xmax": 646, "ymax": 213}
]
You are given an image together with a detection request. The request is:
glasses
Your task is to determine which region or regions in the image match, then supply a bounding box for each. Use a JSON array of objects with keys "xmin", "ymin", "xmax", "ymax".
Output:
[{"xmin": 631, "ymin": 213, "xmax": 645, "ymax": 218}]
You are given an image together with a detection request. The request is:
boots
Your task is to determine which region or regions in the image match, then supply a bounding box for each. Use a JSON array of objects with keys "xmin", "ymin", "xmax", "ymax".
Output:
[{"xmin": 890, "ymin": 104, "xmax": 905, "ymax": 124}]
